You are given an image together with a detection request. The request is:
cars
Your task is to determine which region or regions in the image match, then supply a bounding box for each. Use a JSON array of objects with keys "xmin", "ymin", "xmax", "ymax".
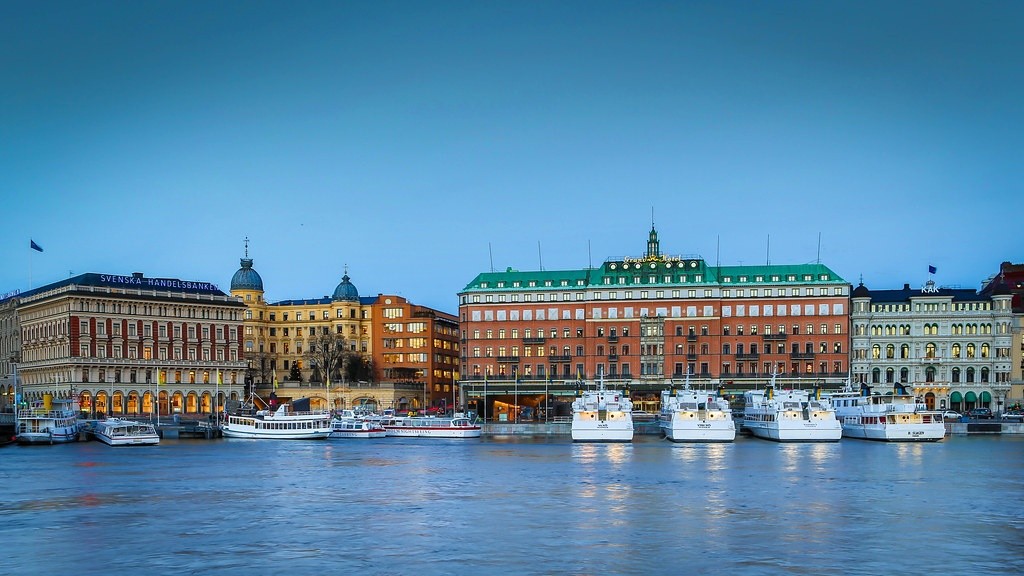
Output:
[
  {"xmin": 1000, "ymin": 411, "xmax": 1024, "ymax": 420},
  {"xmin": 335, "ymin": 409, "xmax": 343, "ymax": 416},
  {"xmin": 934, "ymin": 408, "xmax": 963, "ymax": 419}
]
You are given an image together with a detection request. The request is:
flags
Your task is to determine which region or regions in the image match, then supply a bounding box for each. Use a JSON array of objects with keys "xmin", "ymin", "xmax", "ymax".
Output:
[
  {"xmin": 547, "ymin": 370, "xmax": 552, "ymax": 383},
  {"xmin": 218, "ymin": 371, "xmax": 222, "ymax": 384},
  {"xmin": 517, "ymin": 370, "xmax": 520, "ymax": 383},
  {"xmin": 929, "ymin": 265, "xmax": 936, "ymax": 274},
  {"xmin": 31, "ymin": 240, "xmax": 43, "ymax": 252},
  {"xmin": 157, "ymin": 371, "xmax": 161, "ymax": 384},
  {"xmin": 274, "ymin": 370, "xmax": 278, "ymax": 388},
  {"xmin": 576, "ymin": 369, "xmax": 582, "ymax": 388}
]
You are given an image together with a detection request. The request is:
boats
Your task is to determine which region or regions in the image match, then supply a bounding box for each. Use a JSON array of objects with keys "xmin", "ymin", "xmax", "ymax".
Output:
[
  {"xmin": 94, "ymin": 417, "xmax": 160, "ymax": 447},
  {"xmin": 14, "ymin": 394, "xmax": 84, "ymax": 446},
  {"xmin": 828, "ymin": 372, "xmax": 947, "ymax": 443},
  {"xmin": 219, "ymin": 377, "xmax": 482, "ymax": 440},
  {"xmin": 657, "ymin": 364, "xmax": 737, "ymax": 443},
  {"xmin": 571, "ymin": 364, "xmax": 635, "ymax": 443},
  {"xmin": 742, "ymin": 365, "xmax": 844, "ymax": 444}
]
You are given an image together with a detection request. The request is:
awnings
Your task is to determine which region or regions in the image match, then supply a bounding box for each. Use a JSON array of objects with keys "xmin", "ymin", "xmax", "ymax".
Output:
[
  {"xmin": 951, "ymin": 392, "xmax": 962, "ymax": 402},
  {"xmin": 965, "ymin": 391, "xmax": 977, "ymax": 402},
  {"xmin": 400, "ymin": 398, "xmax": 408, "ymax": 403},
  {"xmin": 980, "ymin": 391, "xmax": 991, "ymax": 402}
]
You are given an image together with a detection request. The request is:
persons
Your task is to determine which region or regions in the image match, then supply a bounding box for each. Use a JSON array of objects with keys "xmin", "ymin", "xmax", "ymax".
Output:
[
  {"xmin": 968, "ymin": 410, "xmax": 972, "ymax": 416},
  {"xmin": 92, "ymin": 410, "xmax": 104, "ymax": 419},
  {"xmin": 1007, "ymin": 400, "xmax": 1021, "ymax": 411},
  {"xmin": 538, "ymin": 412, "xmax": 541, "ymax": 421},
  {"xmin": 109, "ymin": 408, "xmax": 113, "ymax": 416},
  {"xmin": 84, "ymin": 411, "xmax": 88, "ymax": 419}
]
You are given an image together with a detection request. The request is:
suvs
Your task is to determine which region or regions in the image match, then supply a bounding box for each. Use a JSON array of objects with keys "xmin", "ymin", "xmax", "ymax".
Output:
[{"xmin": 965, "ymin": 407, "xmax": 995, "ymax": 420}]
